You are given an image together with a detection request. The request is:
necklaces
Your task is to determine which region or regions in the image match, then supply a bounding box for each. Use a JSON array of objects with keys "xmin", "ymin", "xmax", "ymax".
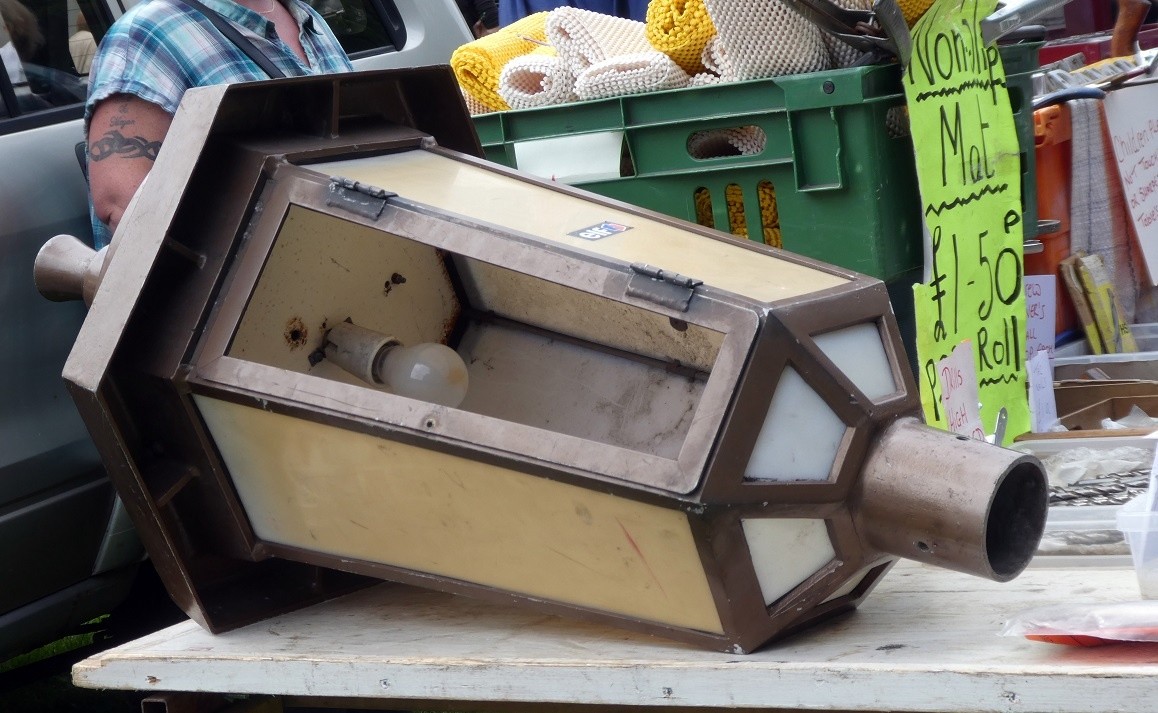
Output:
[{"xmin": 253, "ymin": 0, "xmax": 275, "ymax": 14}]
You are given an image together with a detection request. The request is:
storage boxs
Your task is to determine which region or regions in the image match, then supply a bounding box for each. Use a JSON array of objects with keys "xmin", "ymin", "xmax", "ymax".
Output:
[
  {"xmin": 1007, "ymin": 323, "xmax": 1158, "ymax": 600},
  {"xmin": 469, "ymin": 42, "xmax": 1149, "ymax": 388}
]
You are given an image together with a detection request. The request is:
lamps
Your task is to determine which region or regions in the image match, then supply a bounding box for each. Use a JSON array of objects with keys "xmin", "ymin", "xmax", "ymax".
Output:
[{"xmin": 63, "ymin": 65, "xmax": 1049, "ymax": 655}]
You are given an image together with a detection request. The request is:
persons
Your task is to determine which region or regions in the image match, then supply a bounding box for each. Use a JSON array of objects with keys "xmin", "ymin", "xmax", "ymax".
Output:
[
  {"xmin": 1111, "ymin": 0, "xmax": 1153, "ymax": 59},
  {"xmin": 0, "ymin": 0, "xmax": 81, "ymax": 118},
  {"xmin": 80, "ymin": 0, "xmax": 355, "ymax": 243}
]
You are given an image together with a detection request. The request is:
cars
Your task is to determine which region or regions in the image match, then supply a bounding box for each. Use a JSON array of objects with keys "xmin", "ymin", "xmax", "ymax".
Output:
[{"xmin": 0, "ymin": 1, "xmax": 478, "ymax": 713}]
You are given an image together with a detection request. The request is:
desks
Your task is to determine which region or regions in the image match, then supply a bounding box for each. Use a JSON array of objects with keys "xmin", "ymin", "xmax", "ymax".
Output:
[{"xmin": 74, "ymin": 360, "xmax": 1158, "ymax": 713}]
